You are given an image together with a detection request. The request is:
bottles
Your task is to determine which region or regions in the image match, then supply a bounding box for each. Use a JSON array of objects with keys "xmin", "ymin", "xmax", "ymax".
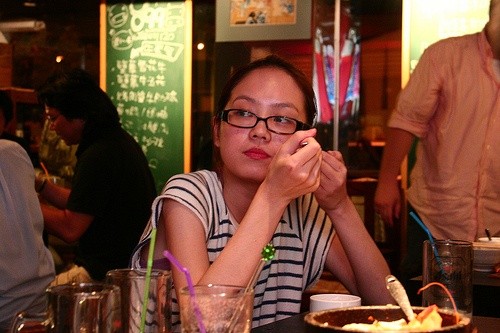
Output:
[{"xmin": 14, "ymin": 129, "xmax": 28, "ymax": 151}]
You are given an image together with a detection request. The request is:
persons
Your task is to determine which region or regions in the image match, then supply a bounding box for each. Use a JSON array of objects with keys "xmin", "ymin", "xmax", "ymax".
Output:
[
  {"xmin": 128, "ymin": 55, "xmax": 397, "ymax": 333},
  {"xmin": 0, "ymin": 67, "xmax": 157, "ymax": 333},
  {"xmin": 376, "ymin": 0, "xmax": 500, "ymax": 307}
]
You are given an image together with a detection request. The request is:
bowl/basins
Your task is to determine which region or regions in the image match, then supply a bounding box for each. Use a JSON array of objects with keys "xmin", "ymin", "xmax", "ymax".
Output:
[
  {"xmin": 470, "ymin": 236, "xmax": 500, "ymax": 273},
  {"xmin": 309, "ymin": 293, "xmax": 363, "ymax": 313},
  {"xmin": 303, "ymin": 304, "xmax": 475, "ymax": 333}
]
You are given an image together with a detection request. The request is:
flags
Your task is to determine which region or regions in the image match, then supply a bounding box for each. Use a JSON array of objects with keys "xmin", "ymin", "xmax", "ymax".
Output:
[{"xmin": 311, "ymin": 34, "xmax": 360, "ymax": 124}]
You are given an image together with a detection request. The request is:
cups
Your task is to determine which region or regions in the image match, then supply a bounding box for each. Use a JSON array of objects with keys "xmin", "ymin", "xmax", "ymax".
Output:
[
  {"xmin": 179, "ymin": 283, "xmax": 253, "ymax": 333},
  {"xmin": 44, "ymin": 282, "xmax": 120, "ymax": 333},
  {"xmin": 11, "ymin": 309, "xmax": 49, "ymax": 333},
  {"xmin": 107, "ymin": 268, "xmax": 173, "ymax": 333},
  {"xmin": 50, "ymin": 177, "xmax": 65, "ymax": 187},
  {"xmin": 422, "ymin": 239, "xmax": 474, "ymax": 321}
]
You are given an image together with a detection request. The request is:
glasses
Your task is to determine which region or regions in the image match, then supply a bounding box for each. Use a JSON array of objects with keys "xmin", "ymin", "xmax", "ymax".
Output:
[
  {"xmin": 219, "ymin": 107, "xmax": 311, "ymax": 135},
  {"xmin": 47, "ymin": 114, "xmax": 61, "ymax": 125}
]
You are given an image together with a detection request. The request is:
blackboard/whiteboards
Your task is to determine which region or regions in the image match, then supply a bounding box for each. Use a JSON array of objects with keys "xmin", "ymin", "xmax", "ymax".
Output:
[{"xmin": 100, "ymin": 0, "xmax": 191, "ymax": 196}]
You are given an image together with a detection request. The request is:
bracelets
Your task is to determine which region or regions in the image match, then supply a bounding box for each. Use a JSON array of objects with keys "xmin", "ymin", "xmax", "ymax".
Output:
[{"xmin": 38, "ymin": 178, "xmax": 47, "ymax": 193}]
